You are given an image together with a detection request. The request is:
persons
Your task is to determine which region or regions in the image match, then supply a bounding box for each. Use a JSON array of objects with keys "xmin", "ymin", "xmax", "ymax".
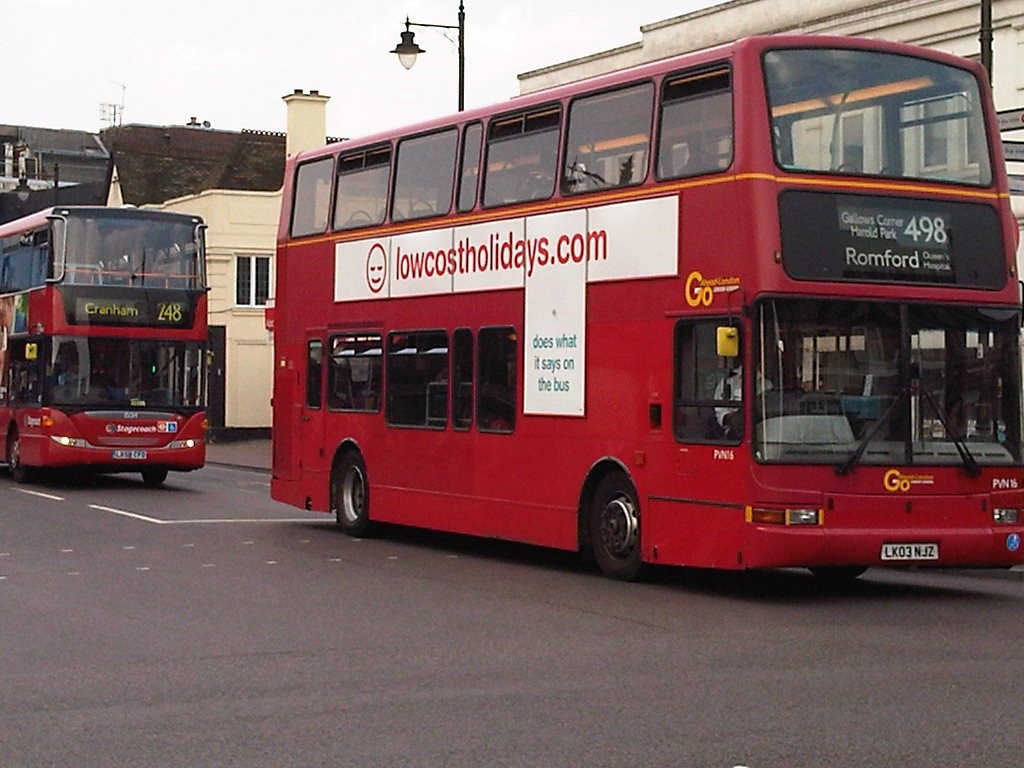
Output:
[
  {"xmin": 0, "ymin": 296, "xmax": 15, "ymax": 333},
  {"xmin": 308, "ymin": 346, "xmax": 514, "ymax": 430},
  {"xmin": 712, "ymin": 353, "xmax": 774, "ymax": 440}
]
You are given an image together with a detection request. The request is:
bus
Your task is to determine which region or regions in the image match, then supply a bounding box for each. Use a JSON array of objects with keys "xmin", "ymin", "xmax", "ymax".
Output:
[
  {"xmin": 271, "ymin": 34, "xmax": 1024, "ymax": 585},
  {"xmin": 1, "ymin": 204, "xmax": 212, "ymax": 483}
]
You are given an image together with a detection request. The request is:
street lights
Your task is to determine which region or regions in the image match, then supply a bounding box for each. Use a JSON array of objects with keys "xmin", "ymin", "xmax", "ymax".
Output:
[
  {"xmin": 11, "ymin": 162, "xmax": 60, "ymax": 207},
  {"xmin": 390, "ymin": 0, "xmax": 467, "ymax": 114}
]
